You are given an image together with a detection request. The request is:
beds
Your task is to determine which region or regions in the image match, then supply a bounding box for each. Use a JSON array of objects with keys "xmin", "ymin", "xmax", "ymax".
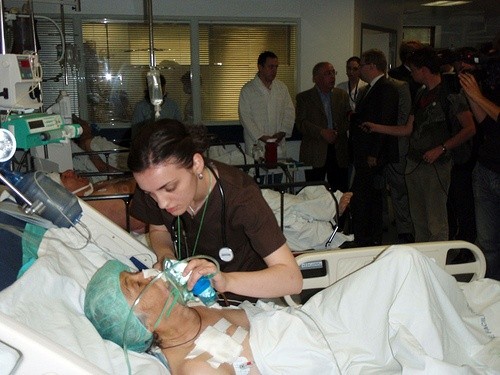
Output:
[{"xmin": 0, "ymin": 140, "xmax": 500, "ymax": 375}]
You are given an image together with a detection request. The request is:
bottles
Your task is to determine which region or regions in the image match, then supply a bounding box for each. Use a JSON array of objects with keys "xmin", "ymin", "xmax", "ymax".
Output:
[{"xmin": 251, "ymin": 143, "xmax": 264, "ymax": 164}]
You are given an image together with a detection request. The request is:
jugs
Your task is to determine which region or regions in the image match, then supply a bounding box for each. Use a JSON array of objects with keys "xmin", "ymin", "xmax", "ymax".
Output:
[{"xmin": 265, "ymin": 139, "xmax": 277, "ymax": 168}]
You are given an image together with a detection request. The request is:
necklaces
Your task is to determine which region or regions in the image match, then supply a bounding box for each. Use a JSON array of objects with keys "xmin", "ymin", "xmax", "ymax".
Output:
[{"xmin": 177, "ymin": 166, "xmax": 212, "ymax": 261}]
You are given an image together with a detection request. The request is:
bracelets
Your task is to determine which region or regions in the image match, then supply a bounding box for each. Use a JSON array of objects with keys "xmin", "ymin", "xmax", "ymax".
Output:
[{"xmin": 441, "ymin": 144, "xmax": 447, "ymax": 153}]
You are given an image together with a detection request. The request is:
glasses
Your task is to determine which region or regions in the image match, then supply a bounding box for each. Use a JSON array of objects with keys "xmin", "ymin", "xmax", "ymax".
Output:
[{"xmin": 318, "ymin": 72, "xmax": 338, "ymax": 76}]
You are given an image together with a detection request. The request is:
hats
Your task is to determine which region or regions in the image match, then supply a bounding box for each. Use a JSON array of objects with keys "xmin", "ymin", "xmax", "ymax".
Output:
[{"xmin": 85, "ymin": 260, "xmax": 155, "ymax": 353}]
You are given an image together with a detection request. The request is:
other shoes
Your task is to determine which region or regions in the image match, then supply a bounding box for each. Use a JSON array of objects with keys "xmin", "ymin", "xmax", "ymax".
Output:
[{"xmin": 400, "ymin": 233, "xmax": 415, "ymax": 244}]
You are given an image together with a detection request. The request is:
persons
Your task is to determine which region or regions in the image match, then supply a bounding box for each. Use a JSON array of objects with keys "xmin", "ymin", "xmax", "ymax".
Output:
[
  {"xmin": 295, "ymin": 61, "xmax": 352, "ymax": 193},
  {"xmin": 238, "ymin": 51, "xmax": 296, "ymax": 192},
  {"xmin": 125, "ymin": 119, "xmax": 303, "ymax": 307},
  {"xmin": 133, "ymin": 74, "xmax": 180, "ymax": 124},
  {"xmin": 82, "ymin": 246, "xmax": 500, "ymax": 375},
  {"xmin": 110, "ymin": 90, "xmax": 133, "ymax": 120},
  {"xmin": 46, "ymin": 170, "xmax": 353, "ymax": 234},
  {"xmin": 70, "ymin": 113, "xmax": 128, "ymax": 173},
  {"xmin": 180, "ymin": 70, "xmax": 210, "ymax": 119},
  {"xmin": 336, "ymin": 39, "xmax": 500, "ymax": 283}
]
